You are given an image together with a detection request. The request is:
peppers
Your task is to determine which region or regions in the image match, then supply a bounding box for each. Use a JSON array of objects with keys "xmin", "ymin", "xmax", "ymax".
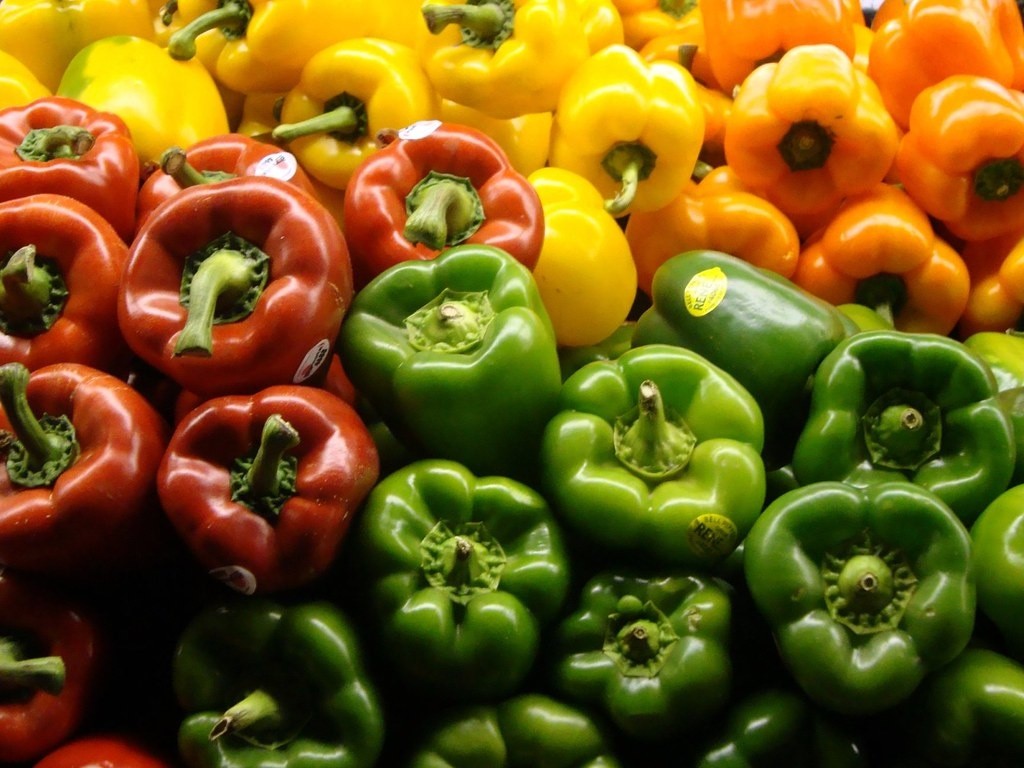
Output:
[{"xmin": 0, "ymin": 0, "xmax": 1024, "ymax": 768}]
